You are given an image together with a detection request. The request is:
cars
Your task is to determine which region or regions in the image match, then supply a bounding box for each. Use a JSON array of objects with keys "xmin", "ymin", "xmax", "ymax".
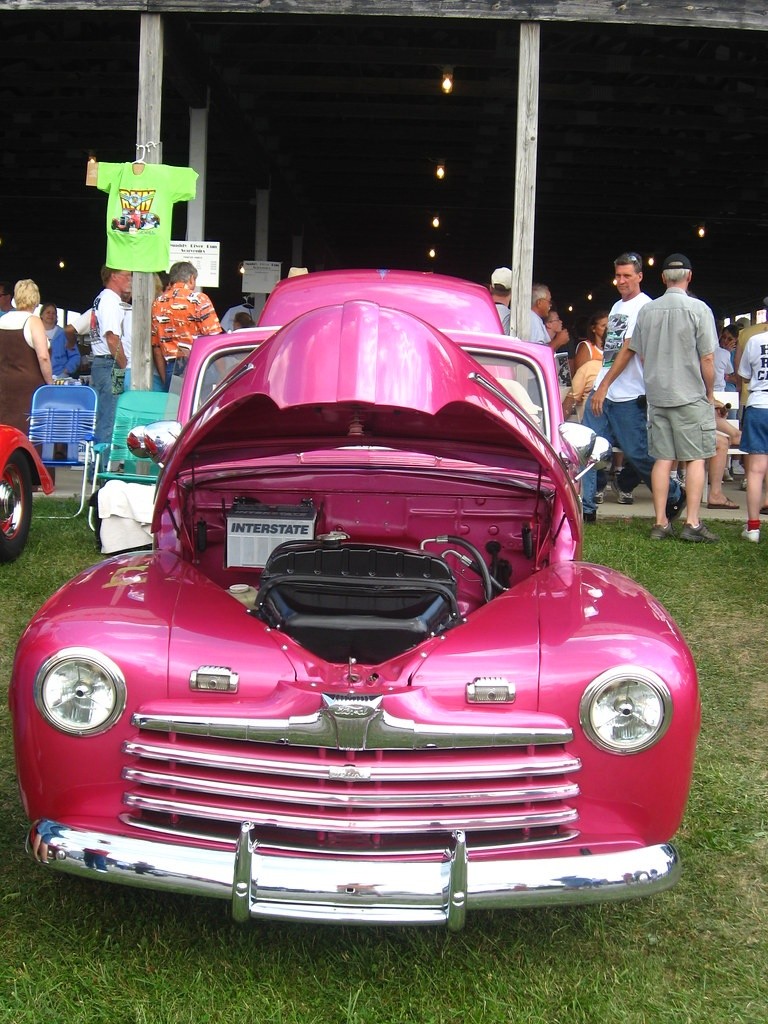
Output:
[
  {"xmin": 9, "ymin": 261, "xmax": 700, "ymax": 935},
  {"xmin": 0, "ymin": 423, "xmax": 55, "ymax": 565}
]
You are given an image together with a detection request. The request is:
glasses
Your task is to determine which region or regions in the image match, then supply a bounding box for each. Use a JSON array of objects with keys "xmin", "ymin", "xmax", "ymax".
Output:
[
  {"xmin": 628, "ymin": 256, "xmax": 640, "ymax": 266},
  {"xmin": 542, "ymin": 298, "xmax": 553, "ymax": 305}
]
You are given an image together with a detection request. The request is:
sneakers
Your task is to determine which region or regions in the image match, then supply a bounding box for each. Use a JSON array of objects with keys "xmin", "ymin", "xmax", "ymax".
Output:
[
  {"xmin": 594, "ymin": 488, "xmax": 607, "ymax": 503},
  {"xmin": 650, "ymin": 520, "xmax": 680, "ymax": 540},
  {"xmin": 665, "ymin": 490, "xmax": 687, "ymax": 522},
  {"xmin": 679, "ymin": 522, "xmax": 720, "ymax": 543},
  {"xmin": 611, "ymin": 477, "xmax": 633, "ymax": 504},
  {"xmin": 741, "ymin": 528, "xmax": 760, "ymax": 543}
]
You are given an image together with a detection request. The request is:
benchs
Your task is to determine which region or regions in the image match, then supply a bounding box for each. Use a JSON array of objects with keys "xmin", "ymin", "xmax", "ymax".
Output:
[{"xmin": 604, "ymin": 391, "xmax": 750, "ymax": 502}]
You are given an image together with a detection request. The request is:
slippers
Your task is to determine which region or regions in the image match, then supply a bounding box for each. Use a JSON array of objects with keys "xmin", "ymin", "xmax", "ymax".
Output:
[{"xmin": 708, "ymin": 497, "xmax": 740, "ymax": 509}]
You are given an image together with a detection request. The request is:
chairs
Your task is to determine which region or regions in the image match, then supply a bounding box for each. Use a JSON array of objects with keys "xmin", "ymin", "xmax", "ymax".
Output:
[
  {"xmin": 28, "ymin": 386, "xmax": 99, "ymax": 519},
  {"xmin": 88, "ymin": 389, "xmax": 185, "ymax": 532}
]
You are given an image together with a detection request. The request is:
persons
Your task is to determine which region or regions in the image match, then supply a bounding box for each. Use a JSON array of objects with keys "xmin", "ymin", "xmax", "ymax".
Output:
[
  {"xmin": 65, "ymin": 267, "xmax": 132, "ymax": 485},
  {"xmin": 39, "ymin": 304, "xmax": 81, "ymax": 459},
  {"xmin": 628, "ymin": 255, "xmax": 718, "ymax": 543},
  {"xmin": 669, "ymin": 298, "xmax": 768, "ymax": 542},
  {"xmin": 531, "ymin": 284, "xmax": 569, "ymax": 354},
  {"xmin": 563, "ymin": 253, "xmax": 687, "ymax": 523},
  {"xmin": 128, "ymin": 432, "xmax": 165, "ymax": 457},
  {"xmin": 0, "ymin": 279, "xmax": 53, "ymax": 490},
  {"xmin": 152, "ymin": 262, "xmax": 256, "ymax": 393},
  {"xmin": 490, "ymin": 267, "xmax": 512, "ymax": 335}
]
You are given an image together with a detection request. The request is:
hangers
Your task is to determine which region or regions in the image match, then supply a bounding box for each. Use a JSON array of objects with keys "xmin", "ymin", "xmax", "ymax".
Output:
[{"xmin": 132, "ymin": 145, "xmax": 147, "ymax": 164}]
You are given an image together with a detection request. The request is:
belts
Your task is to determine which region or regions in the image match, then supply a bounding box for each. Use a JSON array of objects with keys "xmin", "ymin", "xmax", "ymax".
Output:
[{"xmin": 96, "ymin": 355, "xmax": 112, "ymax": 359}]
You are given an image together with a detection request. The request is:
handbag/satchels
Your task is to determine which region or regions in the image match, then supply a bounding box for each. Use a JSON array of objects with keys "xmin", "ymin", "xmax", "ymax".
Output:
[{"xmin": 110, "ymin": 366, "xmax": 126, "ymax": 394}]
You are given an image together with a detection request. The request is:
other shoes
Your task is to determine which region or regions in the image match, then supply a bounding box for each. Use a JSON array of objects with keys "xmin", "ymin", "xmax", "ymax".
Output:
[
  {"xmin": 583, "ymin": 511, "xmax": 596, "ymax": 520},
  {"xmin": 742, "ymin": 480, "xmax": 747, "ymax": 490},
  {"xmin": 723, "ymin": 471, "xmax": 733, "ymax": 481}
]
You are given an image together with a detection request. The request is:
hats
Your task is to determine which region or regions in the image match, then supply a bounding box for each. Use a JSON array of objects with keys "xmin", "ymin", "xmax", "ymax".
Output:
[
  {"xmin": 491, "ymin": 267, "xmax": 512, "ymax": 288},
  {"xmin": 664, "ymin": 253, "xmax": 692, "ymax": 269}
]
